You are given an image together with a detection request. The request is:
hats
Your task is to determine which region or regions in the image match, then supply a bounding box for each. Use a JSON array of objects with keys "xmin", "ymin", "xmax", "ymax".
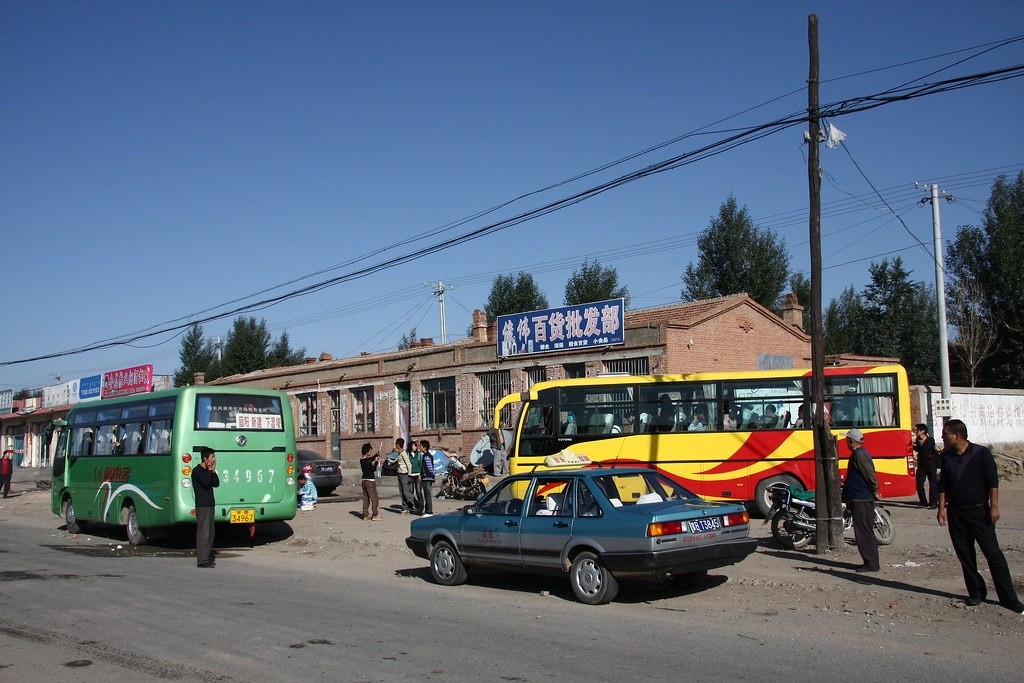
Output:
[{"xmin": 839, "ymin": 429, "xmax": 864, "ymax": 442}]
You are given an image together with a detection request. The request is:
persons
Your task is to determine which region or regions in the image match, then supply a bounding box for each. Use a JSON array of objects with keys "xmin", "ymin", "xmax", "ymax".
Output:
[
  {"xmin": 360, "ymin": 443, "xmax": 384, "ymax": 521},
  {"xmin": 420, "ymin": 440, "xmax": 435, "ymax": 518},
  {"xmin": 611, "ymin": 410, "xmax": 635, "ymax": 433},
  {"xmin": 793, "ymin": 404, "xmax": 804, "ymax": 428},
  {"xmin": 687, "ymin": 406, "xmax": 708, "ymax": 431},
  {"xmin": 937, "ymin": 419, "xmax": 1024, "ymax": 614},
  {"xmin": 297, "ymin": 475, "xmax": 317, "ymax": 508},
  {"xmin": 387, "ymin": 437, "xmax": 419, "ymax": 513},
  {"xmin": 80, "ymin": 424, "xmax": 160, "ymax": 455},
  {"xmin": 192, "ymin": 448, "xmax": 220, "ymax": 568},
  {"xmin": 652, "ymin": 394, "xmax": 674, "ymax": 432},
  {"xmin": 724, "ymin": 401, "xmax": 758, "ymax": 429},
  {"xmin": 406, "ymin": 441, "xmax": 424, "ymax": 513},
  {"xmin": 913, "ymin": 423, "xmax": 940, "ymax": 509},
  {"xmin": 0, "ymin": 451, "xmax": 12, "ymax": 498},
  {"xmin": 487, "ymin": 417, "xmax": 509, "ymax": 476},
  {"xmin": 831, "ymin": 391, "xmax": 856, "ymax": 427},
  {"xmin": 839, "ymin": 428, "xmax": 882, "ymax": 572},
  {"xmin": 777, "ymin": 400, "xmax": 786, "ymax": 416},
  {"xmin": 755, "ymin": 404, "xmax": 778, "ymax": 429}
]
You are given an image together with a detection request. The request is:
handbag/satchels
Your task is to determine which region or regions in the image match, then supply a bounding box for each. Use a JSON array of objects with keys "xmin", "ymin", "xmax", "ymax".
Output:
[{"xmin": 301, "ymin": 495, "xmax": 314, "ymax": 511}]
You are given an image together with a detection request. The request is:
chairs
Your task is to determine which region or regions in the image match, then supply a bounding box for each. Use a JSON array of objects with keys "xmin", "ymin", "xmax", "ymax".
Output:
[
  {"xmin": 582, "ymin": 489, "xmax": 602, "ymax": 516},
  {"xmin": 196, "ymin": 421, "xmax": 236, "ymax": 428},
  {"xmin": 536, "ymin": 493, "xmax": 572, "ymax": 515}
]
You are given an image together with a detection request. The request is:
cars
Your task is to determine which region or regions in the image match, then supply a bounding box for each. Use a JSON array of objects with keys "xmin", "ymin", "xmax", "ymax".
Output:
[
  {"xmin": 405, "ymin": 449, "xmax": 758, "ymax": 605},
  {"xmin": 296, "ymin": 450, "xmax": 342, "ymax": 497}
]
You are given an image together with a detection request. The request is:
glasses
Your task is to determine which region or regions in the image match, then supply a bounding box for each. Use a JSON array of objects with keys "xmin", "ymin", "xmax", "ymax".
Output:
[{"xmin": 943, "ymin": 432, "xmax": 961, "ymax": 437}]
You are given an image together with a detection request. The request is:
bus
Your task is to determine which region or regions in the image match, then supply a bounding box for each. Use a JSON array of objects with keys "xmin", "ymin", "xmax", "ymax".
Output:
[
  {"xmin": 42, "ymin": 386, "xmax": 298, "ymax": 546},
  {"xmin": 494, "ymin": 364, "xmax": 916, "ymax": 520}
]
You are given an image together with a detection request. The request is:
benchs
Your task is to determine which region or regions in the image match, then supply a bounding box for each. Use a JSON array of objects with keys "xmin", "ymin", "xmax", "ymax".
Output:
[
  {"xmin": 564, "ymin": 406, "xmax": 860, "ymax": 434},
  {"xmin": 610, "ymin": 492, "xmax": 663, "ymax": 507},
  {"xmin": 93, "ymin": 429, "xmax": 171, "ymax": 455}
]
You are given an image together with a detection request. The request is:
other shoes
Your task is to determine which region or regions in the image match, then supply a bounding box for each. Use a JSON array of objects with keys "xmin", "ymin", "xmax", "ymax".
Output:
[
  {"xmin": 372, "ymin": 515, "xmax": 383, "ymax": 520},
  {"xmin": 363, "ymin": 515, "xmax": 372, "ymax": 520},
  {"xmin": 967, "ymin": 595, "xmax": 986, "ymax": 605},
  {"xmin": 918, "ymin": 504, "xmax": 928, "ymax": 508},
  {"xmin": 930, "ymin": 504, "xmax": 936, "ymax": 508},
  {"xmin": 400, "ymin": 507, "xmax": 433, "ymax": 517},
  {"xmin": 1008, "ymin": 600, "xmax": 1024, "ymax": 613},
  {"xmin": 856, "ymin": 566, "xmax": 878, "ymax": 572},
  {"xmin": 198, "ymin": 563, "xmax": 214, "ymax": 568}
]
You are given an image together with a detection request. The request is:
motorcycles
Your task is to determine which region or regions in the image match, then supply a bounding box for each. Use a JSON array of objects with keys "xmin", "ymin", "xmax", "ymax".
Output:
[
  {"xmin": 761, "ymin": 485, "xmax": 895, "ymax": 549},
  {"xmin": 440, "ymin": 455, "xmax": 492, "ymax": 500}
]
